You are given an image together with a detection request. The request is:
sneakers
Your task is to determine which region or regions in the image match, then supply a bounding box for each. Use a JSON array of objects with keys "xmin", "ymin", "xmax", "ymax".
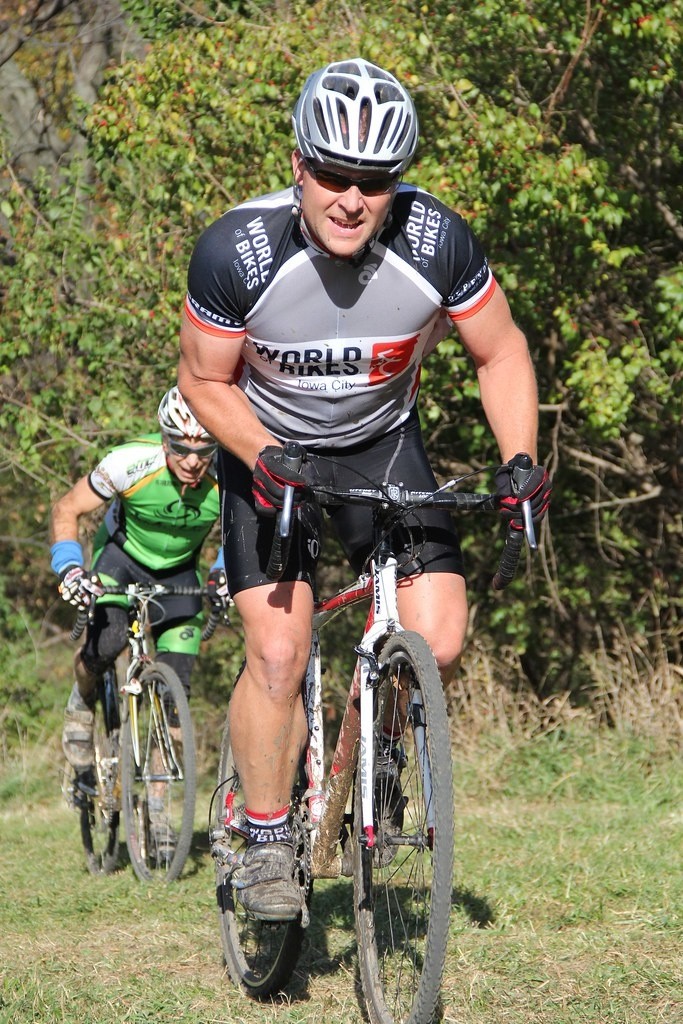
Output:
[
  {"xmin": 370, "ymin": 749, "xmax": 404, "ymax": 869},
  {"xmin": 229, "ymin": 841, "xmax": 310, "ymax": 928}
]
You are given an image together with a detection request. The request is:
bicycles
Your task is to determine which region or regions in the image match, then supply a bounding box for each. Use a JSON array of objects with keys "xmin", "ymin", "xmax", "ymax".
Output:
[
  {"xmin": 58, "ymin": 570, "xmax": 233, "ymax": 884},
  {"xmin": 209, "ymin": 440, "xmax": 537, "ymax": 1024}
]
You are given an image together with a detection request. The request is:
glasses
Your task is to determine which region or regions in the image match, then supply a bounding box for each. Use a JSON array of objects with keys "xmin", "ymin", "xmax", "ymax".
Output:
[
  {"xmin": 301, "ymin": 161, "xmax": 406, "ymax": 193},
  {"xmin": 163, "ymin": 436, "xmax": 218, "ymax": 458}
]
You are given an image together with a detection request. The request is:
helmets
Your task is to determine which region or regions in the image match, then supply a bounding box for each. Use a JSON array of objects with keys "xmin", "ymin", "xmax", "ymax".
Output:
[
  {"xmin": 290, "ymin": 57, "xmax": 421, "ymax": 175},
  {"xmin": 156, "ymin": 385, "xmax": 213, "ymax": 442}
]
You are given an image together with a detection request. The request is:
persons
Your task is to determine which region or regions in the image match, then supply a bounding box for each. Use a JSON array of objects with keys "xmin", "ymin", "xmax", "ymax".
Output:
[
  {"xmin": 178, "ymin": 58, "xmax": 552, "ymax": 921},
  {"xmin": 52, "ymin": 385, "xmax": 236, "ymax": 858}
]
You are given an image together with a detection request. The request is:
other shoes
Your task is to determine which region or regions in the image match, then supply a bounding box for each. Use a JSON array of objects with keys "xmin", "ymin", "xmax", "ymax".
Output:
[
  {"xmin": 61, "ymin": 682, "xmax": 97, "ymax": 772},
  {"xmin": 146, "ymin": 805, "xmax": 175, "ymax": 861}
]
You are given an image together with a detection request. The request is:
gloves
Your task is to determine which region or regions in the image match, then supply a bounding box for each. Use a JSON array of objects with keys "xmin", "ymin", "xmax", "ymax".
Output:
[
  {"xmin": 245, "ymin": 442, "xmax": 326, "ymax": 523},
  {"xmin": 55, "ymin": 564, "xmax": 104, "ymax": 612},
  {"xmin": 487, "ymin": 453, "xmax": 549, "ymax": 528},
  {"xmin": 205, "ymin": 570, "xmax": 238, "ymax": 612}
]
